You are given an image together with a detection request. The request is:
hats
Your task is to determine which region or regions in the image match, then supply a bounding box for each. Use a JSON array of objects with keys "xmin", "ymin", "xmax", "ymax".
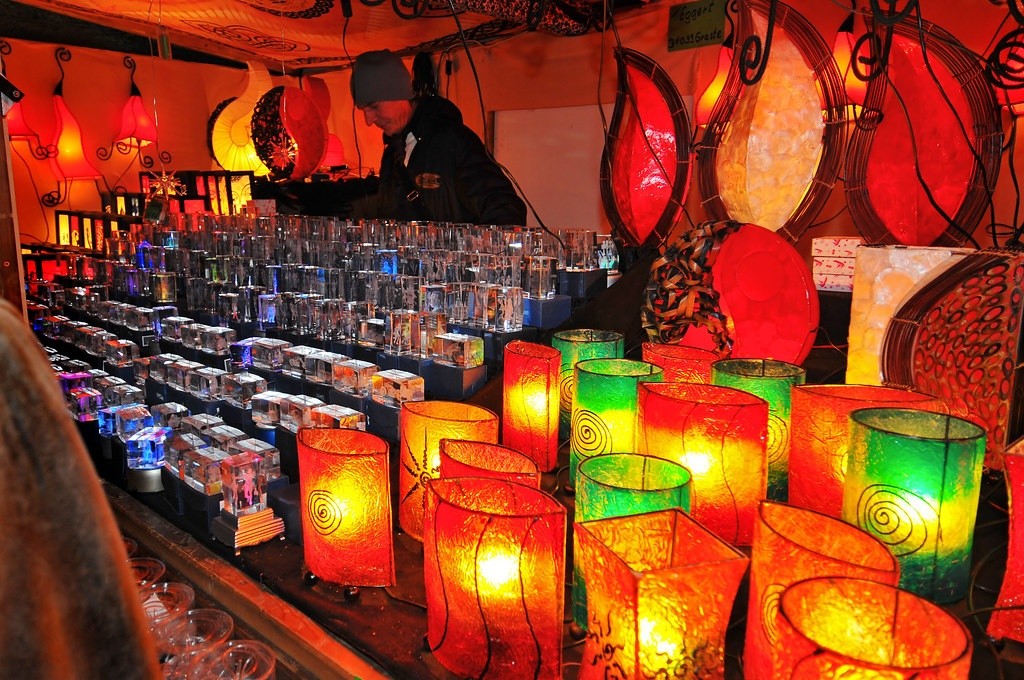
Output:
[{"xmin": 350, "ymin": 49, "xmax": 416, "ymax": 108}]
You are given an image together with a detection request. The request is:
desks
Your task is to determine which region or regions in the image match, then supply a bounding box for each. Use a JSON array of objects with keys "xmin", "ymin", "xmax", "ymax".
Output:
[{"xmin": 92, "ymin": 473, "xmax": 752, "ymax": 679}]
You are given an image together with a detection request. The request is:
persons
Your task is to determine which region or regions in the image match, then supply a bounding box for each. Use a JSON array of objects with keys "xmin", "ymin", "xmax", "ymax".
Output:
[{"xmin": 349, "ymin": 49, "xmax": 527, "ymax": 228}]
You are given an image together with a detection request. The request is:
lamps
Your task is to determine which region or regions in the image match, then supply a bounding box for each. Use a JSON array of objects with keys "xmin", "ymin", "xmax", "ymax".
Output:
[
  {"xmin": 97, "ymin": 53, "xmax": 170, "ymax": 170},
  {"xmin": 23, "ymin": 168, "xmax": 254, "ymax": 297},
  {"xmin": 0, "ymin": 41, "xmax": 60, "ymax": 160},
  {"xmin": 295, "ymin": 0, "xmax": 1024, "ymax": 679},
  {"xmin": 147, "ymin": 0, "xmax": 178, "ymax": 126},
  {"xmin": 42, "ymin": 46, "xmax": 128, "ymax": 207}
]
